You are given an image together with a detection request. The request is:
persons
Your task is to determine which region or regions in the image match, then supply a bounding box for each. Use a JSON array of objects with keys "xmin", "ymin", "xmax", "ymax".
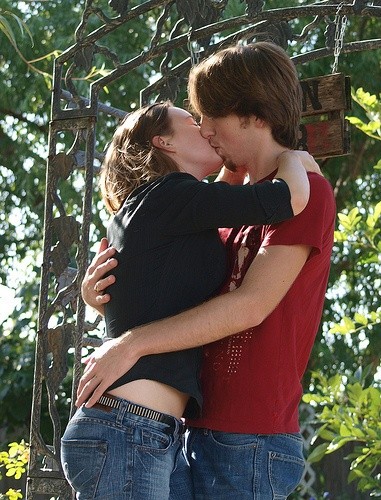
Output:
[
  {"xmin": 60, "ymin": 98, "xmax": 328, "ymax": 500},
  {"xmin": 74, "ymin": 40, "xmax": 338, "ymax": 500}
]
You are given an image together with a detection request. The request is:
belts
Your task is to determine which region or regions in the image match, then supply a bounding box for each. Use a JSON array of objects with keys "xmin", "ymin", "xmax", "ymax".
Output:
[{"xmin": 90, "ymin": 391, "xmax": 176, "ymax": 425}]
